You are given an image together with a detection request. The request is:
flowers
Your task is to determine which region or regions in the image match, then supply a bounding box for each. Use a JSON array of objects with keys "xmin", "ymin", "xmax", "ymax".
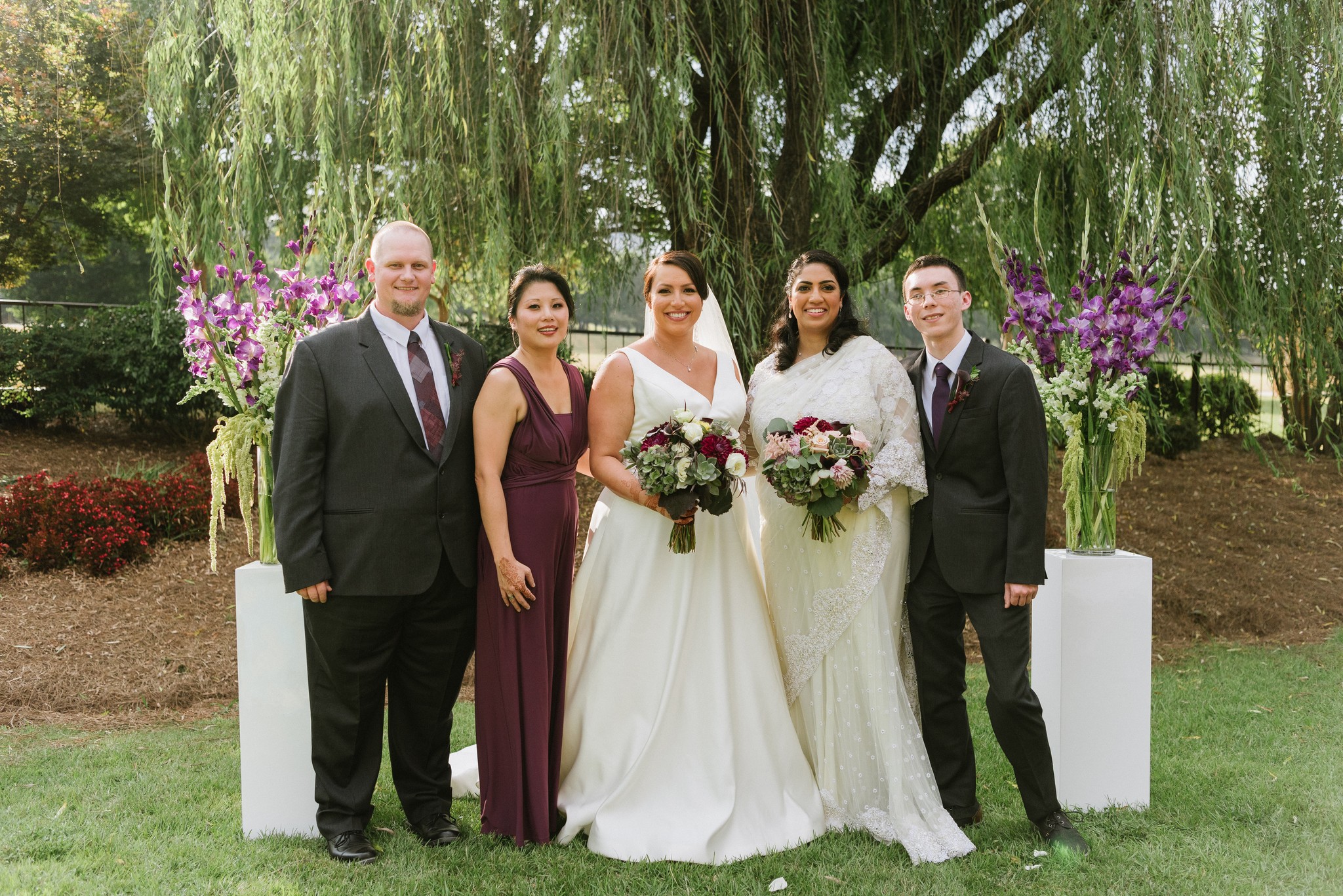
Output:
[
  {"xmin": 763, "ymin": 417, "xmax": 875, "ymax": 544},
  {"xmin": 947, "ymin": 365, "xmax": 980, "ymax": 414},
  {"xmin": 619, "ymin": 397, "xmax": 749, "ymax": 554},
  {"xmin": 170, "ymin": 226, "xmax": 363, "ymax": 572},
  {"xmin": 974, "ymin": 158, "xmax": 1214, "ymax": 550},
  {"xmin": 444, "ymin": 339, "xmax": 464, "ymax": 387}
]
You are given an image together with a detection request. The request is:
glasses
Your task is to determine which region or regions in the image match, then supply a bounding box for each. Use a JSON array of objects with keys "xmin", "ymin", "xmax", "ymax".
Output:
[{"xmin": 905, "ymin": 289, "xmax": 963, "ymax": 305}]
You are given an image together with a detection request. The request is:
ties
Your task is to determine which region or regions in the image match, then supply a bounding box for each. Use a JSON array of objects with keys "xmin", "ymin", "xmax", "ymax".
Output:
[
  {"xmin": 407, "ymin": 331, "xmax": 447, "ymax": 465},
  {"xmin": 931, "ymin": 360, "xmax": 951, "ymax": 451}
]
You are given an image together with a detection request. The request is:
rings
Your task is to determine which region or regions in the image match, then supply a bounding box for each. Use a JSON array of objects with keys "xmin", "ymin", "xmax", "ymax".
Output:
[{"xmin": 508, "ymin": 595, "xmax": 514, "ymax": 600}]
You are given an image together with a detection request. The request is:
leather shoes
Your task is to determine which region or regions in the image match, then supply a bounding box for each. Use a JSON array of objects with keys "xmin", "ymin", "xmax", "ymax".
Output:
[
  {"xmin": 405, "ymin": 813, "xmax": 461, "ymax": 847},
  {"xmin": 326, "ymin": 831, "xmax": 378, "ymax": 866},
  {"xmin": 949, "ymin": 804, "xmax": 979, "ymax": 828},
  {"xmin": 1039, "ymin": 811, "xmax": 1093, "ymax": 864}
]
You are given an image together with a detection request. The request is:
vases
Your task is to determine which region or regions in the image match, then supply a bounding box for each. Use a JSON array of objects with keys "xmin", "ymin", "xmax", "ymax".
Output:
[
  {"xmin": 1065, "ymin": 445, "xmax": 1117, "ymax": 556},
  {"xmin": 256, "ymin": 446, "xmax": 280, "ymax": 565}
]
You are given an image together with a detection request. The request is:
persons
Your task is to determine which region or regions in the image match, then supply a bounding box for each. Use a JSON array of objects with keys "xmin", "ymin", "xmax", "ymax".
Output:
[
  {"xmin": 739, "ymin": 248, "xmax": 978, "ymax": 867},
  {"xmin": 562, "ymin": 247, "xmax": 827, "ymax": 868},
  {"xmin": 898, "ymin": 254, "xmax": 1094, "ymax": 865},
  {"xmin": 469, "ymin": 259, "xmax": 594, "ymax": 847},
  {"xmin": 269, "ymin": 219, "xmax": 492, "ymax": 866}
]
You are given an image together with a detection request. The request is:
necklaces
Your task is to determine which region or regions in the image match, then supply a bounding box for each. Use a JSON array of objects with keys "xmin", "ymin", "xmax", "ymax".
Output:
[
  {"xmin": 653, "ymin": 331, "xmax": 698, "ymax": 372},
  {"xmin": 796, "ymin": 337, "xmax": 808, "ymax": 361}
]
